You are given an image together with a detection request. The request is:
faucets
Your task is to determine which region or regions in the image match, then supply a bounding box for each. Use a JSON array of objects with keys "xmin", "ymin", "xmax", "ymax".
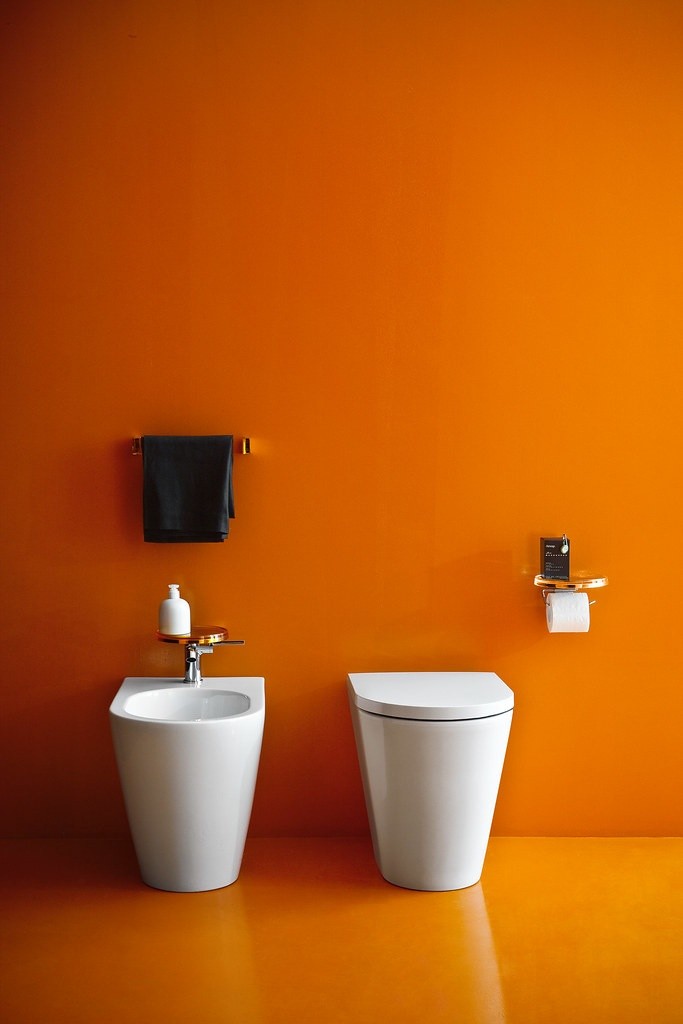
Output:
[{"xmin": 181, "ymin": 638, "xmax": 214, "ymax": 687}]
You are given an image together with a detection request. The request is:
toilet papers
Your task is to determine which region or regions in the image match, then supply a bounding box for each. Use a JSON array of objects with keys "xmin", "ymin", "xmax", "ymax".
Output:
[{"xmin": 543, "ymin": 589, "xmax": 590, "ymax": 634}]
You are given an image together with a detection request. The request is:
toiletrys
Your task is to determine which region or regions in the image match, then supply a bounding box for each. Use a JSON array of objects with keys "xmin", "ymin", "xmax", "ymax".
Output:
[{"xmin": 157, "ymin": 582, "xmax": 191, "ymax": 636}]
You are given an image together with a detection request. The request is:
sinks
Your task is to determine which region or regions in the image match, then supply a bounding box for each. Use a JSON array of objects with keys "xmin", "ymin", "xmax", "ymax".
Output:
[{"xmin": 109, "ymin": 676, "xmax": 268, "ymax": 895}]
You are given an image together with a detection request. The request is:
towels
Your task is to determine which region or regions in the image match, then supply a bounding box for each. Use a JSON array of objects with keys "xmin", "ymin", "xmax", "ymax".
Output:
[{"xmin": 138, "ymin": 433, "xmax": 237, "ymax": 546}]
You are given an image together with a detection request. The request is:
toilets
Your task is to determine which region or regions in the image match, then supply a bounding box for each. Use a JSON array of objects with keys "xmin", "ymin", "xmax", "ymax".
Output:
[{"xmin": 344, "ymin": 668, "xmax": 518, "ymax": 892}]
